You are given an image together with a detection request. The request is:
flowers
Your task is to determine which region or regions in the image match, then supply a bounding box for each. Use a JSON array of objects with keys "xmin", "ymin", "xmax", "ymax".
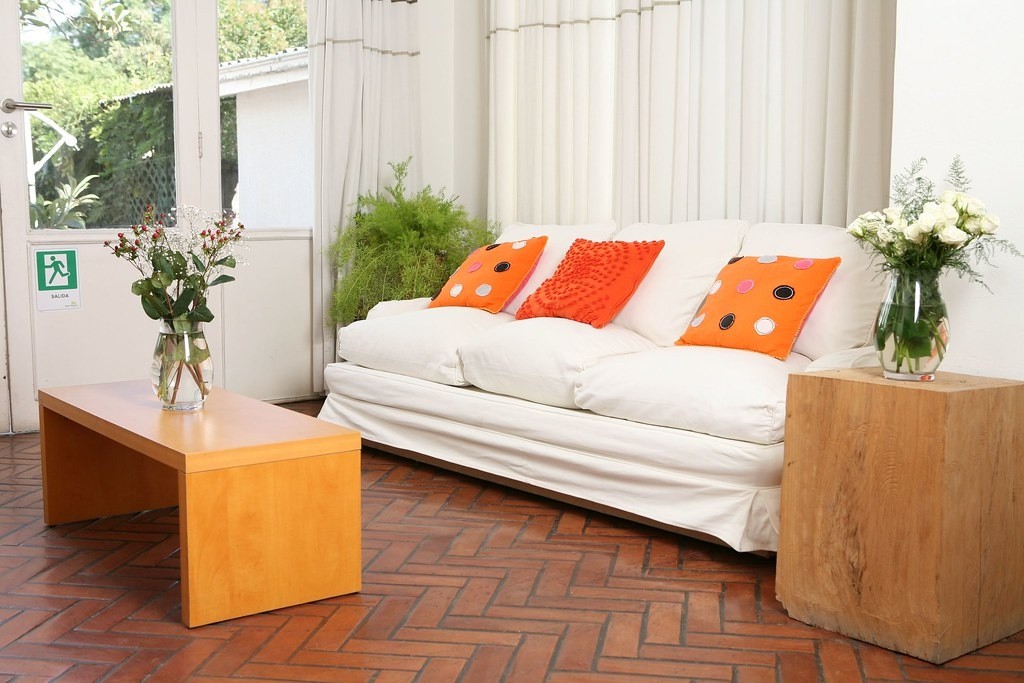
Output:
[
  {"xmin": 104, "ymin": 203, "xmax": 254, "ymax": 404},
  {"xmin": 847, "ymin": 152, "xmax": 1024, "ymax": 375}
]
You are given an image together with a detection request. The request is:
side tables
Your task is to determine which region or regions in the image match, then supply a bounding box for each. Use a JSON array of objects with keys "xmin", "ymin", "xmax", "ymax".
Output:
[{"xmin": 775, "ymin": 367, "xmax": 1023, "ymax": 665}]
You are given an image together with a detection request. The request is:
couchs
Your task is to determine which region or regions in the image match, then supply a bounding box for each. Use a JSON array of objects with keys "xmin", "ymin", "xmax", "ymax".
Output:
[{"xmin": 317, "ymin": 222, "xmax": 893, "ymax": 560}]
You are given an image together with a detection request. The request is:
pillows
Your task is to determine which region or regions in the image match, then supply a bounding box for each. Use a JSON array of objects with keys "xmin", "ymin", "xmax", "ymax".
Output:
[
  {"xmin": 674, "ymin": 255, "xmax": 842, "ymax": 361},
  {"xmin": 515, "ymin": 238, "xmax": 665, "ymax": 328},
  {"xmin": 426, "ymin": 236, "xmax": 548, "ymax": 313}
]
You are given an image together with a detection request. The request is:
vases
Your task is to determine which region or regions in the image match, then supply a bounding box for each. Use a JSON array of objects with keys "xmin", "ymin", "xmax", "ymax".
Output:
[
  {"xmin": 149, "ymin": 318, "xmax": 215, "ymax": 413},
  {"xmin": 874, "ymin": 266, "xmax": 950, "ymax": 381}
]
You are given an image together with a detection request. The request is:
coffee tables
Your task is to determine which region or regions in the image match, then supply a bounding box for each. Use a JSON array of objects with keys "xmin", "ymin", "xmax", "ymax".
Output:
[{"xmin": 38, "ymin": 377, "xmax": 362, "ymax": 628}]
